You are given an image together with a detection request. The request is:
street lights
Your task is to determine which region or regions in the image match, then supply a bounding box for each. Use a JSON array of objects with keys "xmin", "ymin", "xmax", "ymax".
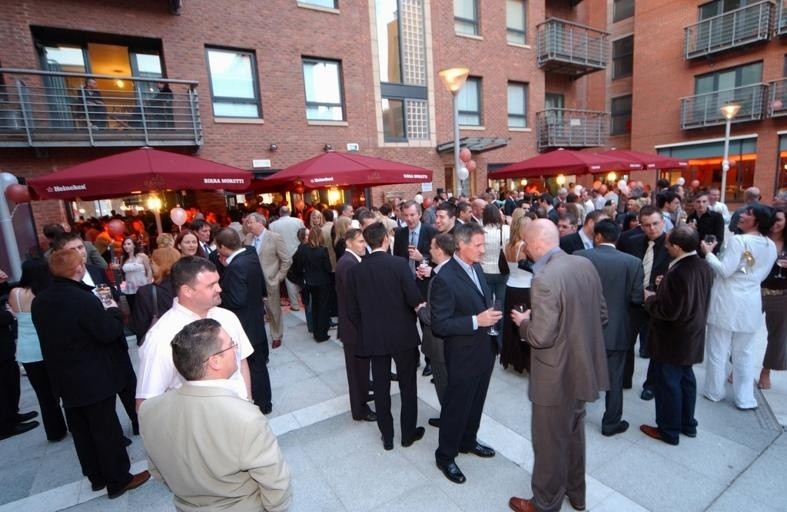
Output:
[
  {"xmin": 438, "ymin": 65, "xmax": 471, "ymax": 196},
  {"xmin": 714, "ymin": 104, "xmax": 741, "ymax": 203}
]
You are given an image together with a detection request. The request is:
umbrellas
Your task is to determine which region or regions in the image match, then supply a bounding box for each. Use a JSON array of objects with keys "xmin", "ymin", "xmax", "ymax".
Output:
[
  {"xmin": 27, "ymin": 147, "xmax": 252, "ymax": 233},
  {"xmin": 600, "ymin": 147, "xmax": 689, "ymax": 170},
  {"xmin": 489, "ymin": 148, "xmax": 630, "ymax": 179},
  {"xmin": 260, "ymin": 144, "xmax": 434, "ymax": 185}
]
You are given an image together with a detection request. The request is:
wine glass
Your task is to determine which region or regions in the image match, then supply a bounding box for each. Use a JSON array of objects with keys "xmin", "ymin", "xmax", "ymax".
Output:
[
  {"xmin": 483, "ymin": 299, "xmax": 502, "ymax": 336},
  {"xmin": 94, "ymin": 282, "xmax": 110, "ymax": 305},
  {"xmin": 772, "ymin": 250, "xmax": 785, "ymax": 279}
]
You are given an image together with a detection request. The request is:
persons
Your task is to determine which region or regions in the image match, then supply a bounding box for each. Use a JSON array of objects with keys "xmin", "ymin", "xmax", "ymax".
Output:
[
  {"xmin": 135, "ymin": 256, "xmax": 256, "ymax": 415},
  {"xmin": 245, "ymin": 181, "xmax": 785, "ymax": 450},
  {"xmin": 510, "ymin": 217, "xmax": 614, "ymax": 512},
  {"xmin": 77, "ymin": 78, "xmax": 107, "ymax": 130},
  {"xmin": 125, "ymin": 78, "xmax": 174, "ymax": 130},
  {"xmin": 430, "ymin": 221, "xmax": 505, "ymax": 483},
  {"xmin": 0, "ymin": 208, "xmax": 273, "ymax": 499},
  {"xmin": 138, "ymin": 319, "xmax": 294, "ymax": 510}
]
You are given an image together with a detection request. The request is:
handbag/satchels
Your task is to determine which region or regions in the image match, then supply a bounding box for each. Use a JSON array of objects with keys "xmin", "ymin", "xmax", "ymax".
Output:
[
  {"xmin": 717, "ymin": 250, "xmax": 754, "ymax": 274},
  {"xmin": 497, "ymin": 248, "xmax": 510, "ymax": 277}
]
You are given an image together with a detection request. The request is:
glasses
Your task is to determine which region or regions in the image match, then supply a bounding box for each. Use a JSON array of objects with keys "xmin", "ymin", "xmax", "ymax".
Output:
[{"xmin": 202, "ymin": 340, "xmax": 238, "ymax": 363}]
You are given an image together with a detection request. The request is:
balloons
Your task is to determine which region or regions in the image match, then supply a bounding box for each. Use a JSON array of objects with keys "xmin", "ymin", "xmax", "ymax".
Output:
[{"xmin": 0, "ymin": 172, "xmax": 26, "ymax": 202}]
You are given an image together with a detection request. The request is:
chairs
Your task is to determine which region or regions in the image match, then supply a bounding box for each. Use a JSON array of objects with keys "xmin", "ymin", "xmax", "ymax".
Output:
[
  {"xmin": 62, "ymin": 93, "xmax": 90, "ymax": 128},
  {"xmin": 149, "ymin": 99, "xmax": 176, "ymax": 129}
]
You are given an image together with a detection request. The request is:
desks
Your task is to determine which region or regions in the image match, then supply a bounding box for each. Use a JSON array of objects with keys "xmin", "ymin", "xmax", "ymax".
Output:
[{"xmin": 107, "ymin": 110, "xmax": 138, "ymax": 131}]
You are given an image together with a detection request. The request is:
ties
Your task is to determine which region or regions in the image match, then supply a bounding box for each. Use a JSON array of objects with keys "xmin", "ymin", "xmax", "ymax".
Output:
[
  {"xmin": 641, "ymin": 241, "xmax": 654, "ymax": 289},
  {"xmin": 204, "ymin": 244, "xmax": 208, "ymax": 254},
  {"xmin": 407, "ymin": 231, "xmax": 417, "ymax": 280},
  {"xmin": 467, "ymin": 268, "xmax": 477, "ymax": 286},
  {"xmin": 253, "ymin": 238, "xmax": 260, "ymax": 253}
]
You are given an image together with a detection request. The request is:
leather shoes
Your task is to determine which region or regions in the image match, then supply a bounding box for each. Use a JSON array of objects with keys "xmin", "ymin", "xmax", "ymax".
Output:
[
  {"xmin": 436, "ymin": 457, "xmax": 464, "ymax": 483},
  {"xmin": 10, "ymin": 410, "xmax": 38, "ymax": 435},
  {"xmin": 472, "ymin": 442, "xmax": 495, "ymax": 457},
  {"xmin": 601, "ymin": 419, "xmax": 627, "ymax": 435},
  {"xmin": 640, "ymin": 387, "xmax": 653, "ymax": 400},
  {"xmin": 271, "ymin": 338, "xmax": 281, "ymax": 348},
  {"xmin": 351, "ymin": 364, "xmax": 439, "ymax": 448},
  {"xmin": 639, "ymin": 423, "xmax": 664, "ymax": 440},
  {"xmin": 509, "ymin": 496, "xmax": 540, "ymax": 511},
  {"xmin": 108, "ymin": 470, "xmax": 151, "ymax": 496}
]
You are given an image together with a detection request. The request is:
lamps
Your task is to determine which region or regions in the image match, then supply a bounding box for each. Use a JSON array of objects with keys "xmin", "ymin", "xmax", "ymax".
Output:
[
  {"xmin": 269, "ymin": 143, "xmax": 277, "ymax": 152},
  {"xmin": 325, "ymin": 144, "xmax": 334, "ymax": 152}
]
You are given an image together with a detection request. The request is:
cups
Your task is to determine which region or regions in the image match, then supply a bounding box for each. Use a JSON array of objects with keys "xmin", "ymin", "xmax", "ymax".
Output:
[
  {"xmin": 408, "ymin": 242, "xmax": 416, "ymax": 250},
  {"xmin": 703, "ymin": 234, "xmax": 716, "ymax": 247},
  {"xmin": 418, "ymin": 259, "xmax": 429, "ymax": 268},
  {"xmin": 511, "ymin": 303, "xmax": 534, "ymax": 343}
]
